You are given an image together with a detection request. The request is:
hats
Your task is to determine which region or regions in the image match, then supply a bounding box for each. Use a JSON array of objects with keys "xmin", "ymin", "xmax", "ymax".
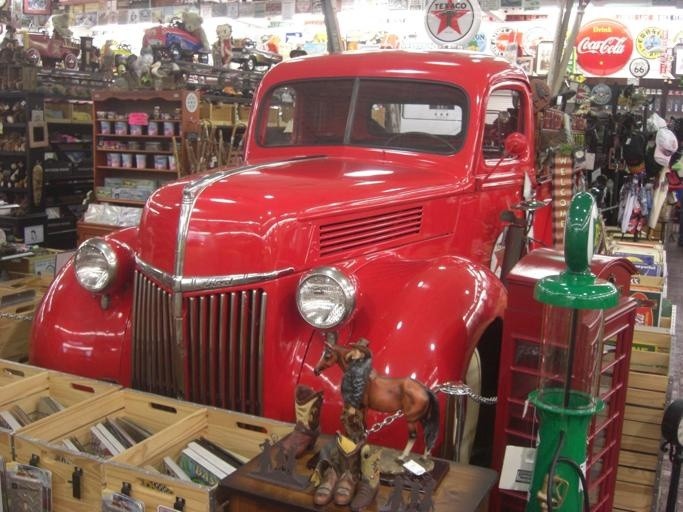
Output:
[
  {"xmin": 653, "ymin": 128, "xmax": 678, "ymax": 167},
  {"xmin": 623, "ymin": 134, "xmax": 646, "ymax": 174}
]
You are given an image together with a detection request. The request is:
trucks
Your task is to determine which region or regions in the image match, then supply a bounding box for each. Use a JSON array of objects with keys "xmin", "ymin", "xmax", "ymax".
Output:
[{"xmin": 29, "ymin": 49, "xmax": 555, "ymax": 460}]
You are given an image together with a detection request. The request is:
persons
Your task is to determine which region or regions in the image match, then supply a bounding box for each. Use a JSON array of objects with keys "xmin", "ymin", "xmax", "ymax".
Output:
[
  {"xmin": 540, "ymin": 47, "xmax": 551, "ymax": 70},
  {"xmin": 27, "ymin": 230, "xmax": 38, "ymax": 243}
]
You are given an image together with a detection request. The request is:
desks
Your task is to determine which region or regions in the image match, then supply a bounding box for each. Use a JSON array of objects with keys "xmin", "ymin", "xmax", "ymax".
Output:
[{"xmin": 218, "ymin": 429, "xmax": 501, "ymax": 512}]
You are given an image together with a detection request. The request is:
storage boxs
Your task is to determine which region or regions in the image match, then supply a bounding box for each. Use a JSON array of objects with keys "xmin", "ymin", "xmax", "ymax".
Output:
[{"xmin": 0, "ymin": 360, "xmax": 296, "ymax": 512}]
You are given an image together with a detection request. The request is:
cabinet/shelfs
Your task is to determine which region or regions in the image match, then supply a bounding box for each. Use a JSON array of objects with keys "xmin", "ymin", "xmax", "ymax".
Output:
[{"xmin": 0, "ymin": 87, "xmax": 294, "ymax": 250}]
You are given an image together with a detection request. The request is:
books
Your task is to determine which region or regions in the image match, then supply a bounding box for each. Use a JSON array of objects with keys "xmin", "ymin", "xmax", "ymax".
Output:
[
  {"xmin": 0, "ymin": 393, "xmax": 253, "ymax": 489},
  {"xmin": 606, "ymin": 239, "xmax": 667, "ymax": 352}
]
